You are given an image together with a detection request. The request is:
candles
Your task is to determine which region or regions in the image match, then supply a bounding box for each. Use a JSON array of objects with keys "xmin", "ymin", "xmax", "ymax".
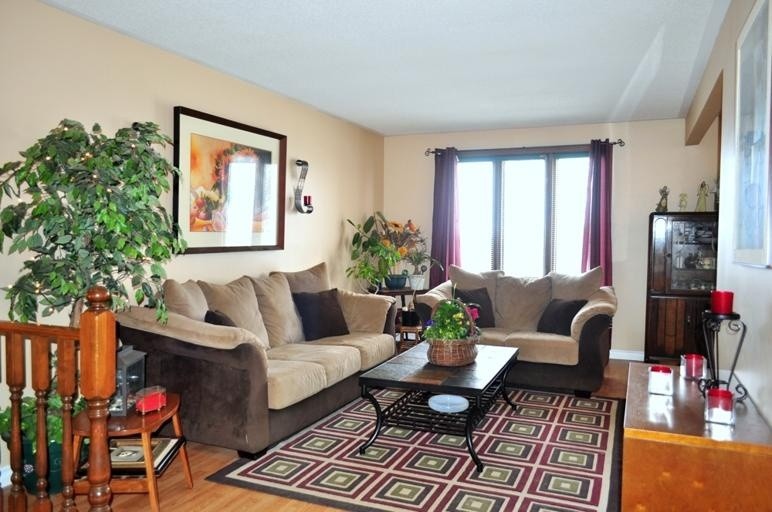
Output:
[
  {"xmin": 303, "ymin": 195, "xmax": 311, "ymax": 204},
  {"xmin": 650, "ymin": 365, "xmax": 672, "ymax": 375}
]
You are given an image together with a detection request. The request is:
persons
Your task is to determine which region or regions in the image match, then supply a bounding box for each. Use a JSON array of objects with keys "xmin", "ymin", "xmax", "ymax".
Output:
[
  {"xmin": 693, "ymin": 181, "xmax": 710, "ymax": 211},
  {"xmin": 678, "ymin": 192, "xmax": 688, "ymax": 212},
  {"xmin": 654, "ymin": 186, "xmax": 669, "ymax": 211}
]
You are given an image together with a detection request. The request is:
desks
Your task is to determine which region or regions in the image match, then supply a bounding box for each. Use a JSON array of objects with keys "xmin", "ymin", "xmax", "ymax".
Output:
[
  {"xmin": 69, "ymin": 391, "xmax": 196, "ymax": 511},
  {"xmin": 366, "ymin": 285, "xmax": 432, "ymax": 341}
]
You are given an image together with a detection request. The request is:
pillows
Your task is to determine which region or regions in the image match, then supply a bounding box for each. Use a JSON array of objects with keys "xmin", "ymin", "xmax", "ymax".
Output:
[
  {"xmin": 451, "ymin": 285, "xmax": 496, "ymax": 329},
  {"xmin": 534, "ymin": 296, "xmax": 589, "ymax": 337},
  {"xmin": 292, "ymin": 285, "xmax": 351, "ymax": 342},
  {"xmin": 204, "ymin": 308, "xmax": 237, "ymax": 328}
]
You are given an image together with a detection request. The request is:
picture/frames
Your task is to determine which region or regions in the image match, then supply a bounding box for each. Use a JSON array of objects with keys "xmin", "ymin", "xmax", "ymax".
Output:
[
  {"xmin": 170, "ymin": 102, "xmax": 289, "ymax": 257},
  {"xmin": 729, "ymin": 1, "xmax": 770, "ymax": 275},
  {"xmin": 76, "ymin": 434, "xmax": 187, "ymax": 478}
]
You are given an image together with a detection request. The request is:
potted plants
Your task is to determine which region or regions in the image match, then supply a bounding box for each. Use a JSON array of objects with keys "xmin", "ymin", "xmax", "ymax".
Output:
[
  {"xmin": 706, "ymin": 387, "xmax": 732, "ymax": 412},
  {"xmin": 0, "ymin": 391, "xmax": 93, "ymax": 501},
  {"xmin": 682, "ymin": 352, "xmax": 703, "ymax": 378},
  {"xmin": 401, "ymin": 256, "xmax": 446, "ymax": 326},
  {"xmin": 403, "ymin": 250, "xmax": 428, "ymax": 291},
  {"xmin": 0, "ymin": 113, "xmax": 203, "ymax": 462},
  {"xmin": 708, "ymin": 288, "xmax": 735, "ymax": 316}
]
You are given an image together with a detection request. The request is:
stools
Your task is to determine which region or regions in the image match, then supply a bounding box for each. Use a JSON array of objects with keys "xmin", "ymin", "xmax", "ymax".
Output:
[{"xmin": 399, "ymin": 325, "xmax": 423, "ymax": 354}]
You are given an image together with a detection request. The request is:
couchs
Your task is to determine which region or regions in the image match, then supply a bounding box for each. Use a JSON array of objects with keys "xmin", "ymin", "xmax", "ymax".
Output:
[
  {"xmin": 104, "ymin": 257, "xmax": 403, "ymax": 460},
  {"xmin": 412, "ymin": 263, "xmax": 618, "ymax": 400}
]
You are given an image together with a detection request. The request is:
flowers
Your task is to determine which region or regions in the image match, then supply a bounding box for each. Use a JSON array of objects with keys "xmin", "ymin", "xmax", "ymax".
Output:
[
  {"xmin": 346, "ymin": 209, "xmax": 429, "ymax": 275},
  {"xmin": 420, "ymin": 282, "xmax": 482, "ymax": 340}
]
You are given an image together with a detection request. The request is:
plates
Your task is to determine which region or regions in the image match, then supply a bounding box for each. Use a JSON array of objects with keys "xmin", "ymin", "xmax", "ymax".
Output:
[{"xmin": 428, "ymin": 394, "xmax": 469, "ymax": 413}]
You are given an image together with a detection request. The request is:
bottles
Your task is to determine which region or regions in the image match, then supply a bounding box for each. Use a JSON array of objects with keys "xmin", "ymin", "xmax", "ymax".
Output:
[{"xmin": 674, "ymin": 221, "xmax": 716, "ymax": 290}]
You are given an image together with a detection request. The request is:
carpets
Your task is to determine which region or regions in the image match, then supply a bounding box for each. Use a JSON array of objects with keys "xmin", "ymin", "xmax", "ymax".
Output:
[{"xmin": 204, "ymin": 384, "xmax": 626, "ymax": 512}]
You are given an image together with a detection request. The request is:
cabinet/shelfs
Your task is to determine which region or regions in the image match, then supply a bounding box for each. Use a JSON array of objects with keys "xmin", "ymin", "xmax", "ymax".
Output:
[
  {"xmin": 619, "ymin": 359, "xmax": 771, "ymax": 512},
  {"xmin": 642, "ymin": 209, "xmax": 721, "ymax": 365}
]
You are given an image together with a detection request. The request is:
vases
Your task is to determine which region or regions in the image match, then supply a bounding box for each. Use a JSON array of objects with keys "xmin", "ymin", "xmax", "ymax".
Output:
[{"xmin": 383, "ymin": 274, "xmax": 407, "ymax": 289}]
